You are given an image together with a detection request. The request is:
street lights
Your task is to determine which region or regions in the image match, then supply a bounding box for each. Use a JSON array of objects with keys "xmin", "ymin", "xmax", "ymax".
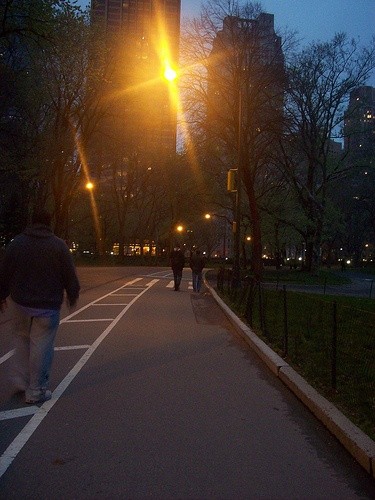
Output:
[{"xmin": 158, "ymin": 63, "xmax": 245, "ymax": 290}]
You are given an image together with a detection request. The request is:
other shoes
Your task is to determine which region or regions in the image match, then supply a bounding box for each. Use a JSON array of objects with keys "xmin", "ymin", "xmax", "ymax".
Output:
[
  {"xmin": 193, "ymin": 288, "xmax": 199, "ymax": 292},
  {"xmin": 24, "ymin": 389, "xmax": 52, "ymax": 404},
  {"xmin": 16, "ymin": 383, "xmax": 29, "ymax": 391},
  {"xmin": 174, "ymin": 285, "xmax": 179, "ymax": 291}
]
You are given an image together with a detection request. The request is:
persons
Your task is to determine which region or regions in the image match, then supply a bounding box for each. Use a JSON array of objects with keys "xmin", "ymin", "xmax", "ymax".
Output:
[
  {"xmin": 0, "ymin": 210, "xmax": 80, "ymax": 404},
  {"xmin": 171, "ymin": 249, "xmax": 185, "ymax": 292},
  {"xmin": 341, "ymin": 257, "xmax": 347, "ymax": 272},
  {"xmin": 188, "ymin": 251, "xmax": 204, "ymax": 293}
]
